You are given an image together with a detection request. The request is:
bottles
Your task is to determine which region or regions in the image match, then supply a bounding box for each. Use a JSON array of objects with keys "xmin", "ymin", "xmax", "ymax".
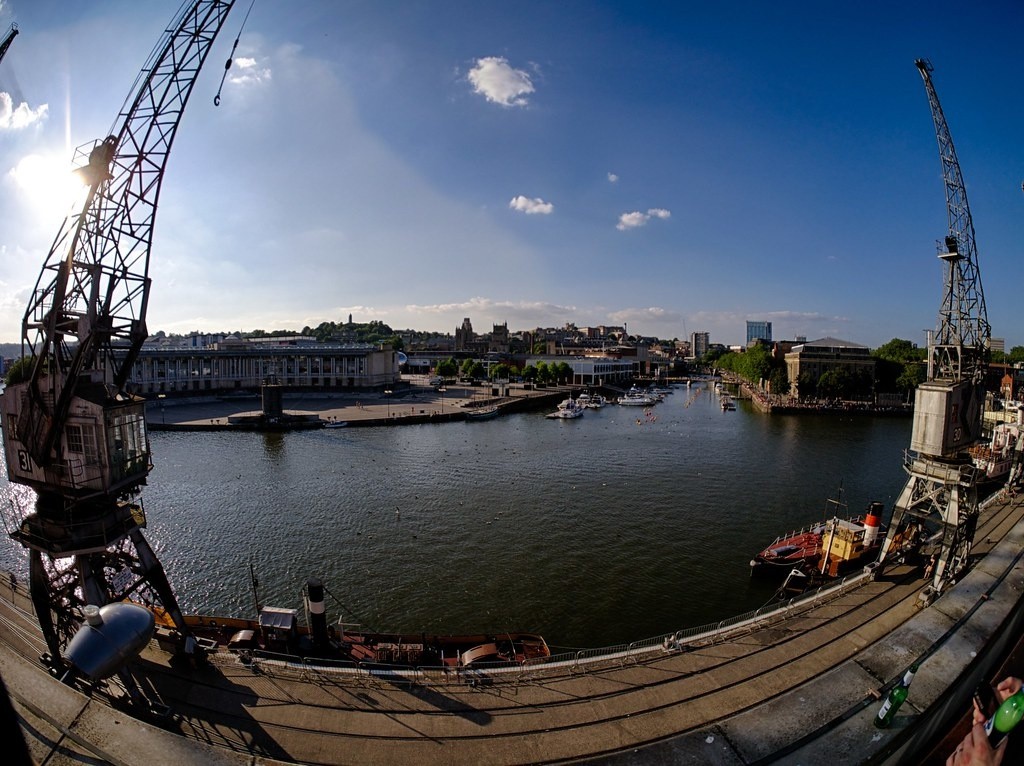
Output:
[
  {"xmin": 981, "ymin": 683, "xmax": 1023, "ymax": 750},
  {"xmin": 873, "ymin": 663, "xmax": 919, "ymax": 729}
]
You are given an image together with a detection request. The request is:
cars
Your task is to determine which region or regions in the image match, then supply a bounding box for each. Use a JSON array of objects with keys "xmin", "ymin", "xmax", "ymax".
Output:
[{"xmin": 430, "ymin": 376, "xmax": 443, "ymax": 385}]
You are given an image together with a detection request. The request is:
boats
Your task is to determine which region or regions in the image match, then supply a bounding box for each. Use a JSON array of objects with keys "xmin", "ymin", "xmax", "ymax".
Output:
[
  {"xmin": 716, "ymin": 383, "xmax": 730, "ymax": 397},
  {"xmin": 545, "ymin": 388, "xmax": 585, "ymax": 418},
  {"xmin": 749, "ymin": 477, "xmax": 925, "ymax": 587},
  {"xmin": 119, "ymin": 561, "xmax": 549, "ymax": 673},
  {"xmin": 322, "ymin": 421, "xmax": 348, "ymax": 428},
  {"xmin": 586, "ymin": 390, "xmax": 606, "ymax": 408},
  {"xmin": 719, "ymin": 395, "xmax": 736, "ymax": 411},
  {"xmin": 576, "ymin": 389, "xmax": 592, "ymax": 409},
  {"xmin": 617, "ymin": 382, "xmax": 671, "ymax": 406},
  {"xmin": 464, "ymin": 403, "xmax": 498, "ymax": 421}
]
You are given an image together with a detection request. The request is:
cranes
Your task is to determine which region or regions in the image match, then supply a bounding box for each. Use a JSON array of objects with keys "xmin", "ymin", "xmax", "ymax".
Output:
[
  {"xmin": 902, "ymin": 55, "xmax": 1019, "ymax": 488},
  {"xmin": 1, "ymin": 0, "xmax": 255, "ymax": 503}
]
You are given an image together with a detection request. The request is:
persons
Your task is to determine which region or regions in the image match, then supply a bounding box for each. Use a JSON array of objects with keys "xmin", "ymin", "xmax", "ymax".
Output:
[
  {"xmin": 1009, "ymin": 489, "xmax": 1018, "ymax": 507},
  {"xmin": 943, "ymin": 676, "xmax": 1024, "ymax": 766},
  {"xmin": 8, "ymin": 573, "xmax": 19, "ymax": 592}
]
